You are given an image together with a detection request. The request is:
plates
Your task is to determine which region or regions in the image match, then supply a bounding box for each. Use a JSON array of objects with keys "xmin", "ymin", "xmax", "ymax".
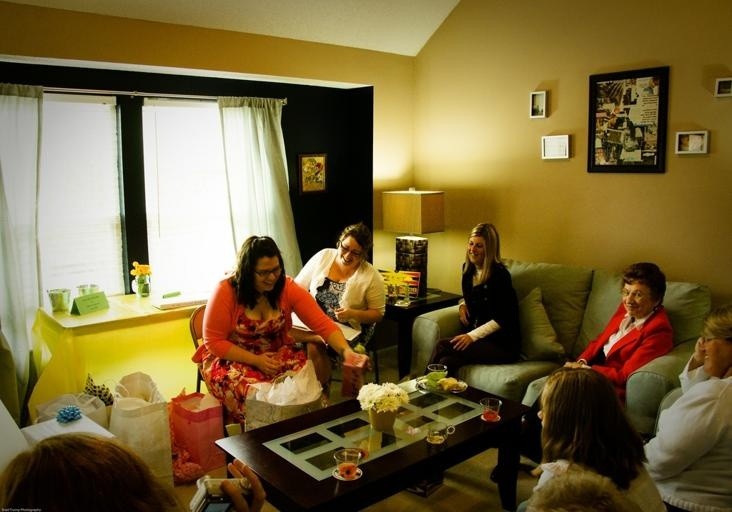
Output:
[{"xmin": 416, "ymin": 376, "xmax": 468, "ymax": 395}]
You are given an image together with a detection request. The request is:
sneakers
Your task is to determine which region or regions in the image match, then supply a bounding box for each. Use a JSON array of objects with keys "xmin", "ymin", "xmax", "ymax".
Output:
[{"xmin": 521, "ymin": 408, "xmax": 541, "ymax": 425}]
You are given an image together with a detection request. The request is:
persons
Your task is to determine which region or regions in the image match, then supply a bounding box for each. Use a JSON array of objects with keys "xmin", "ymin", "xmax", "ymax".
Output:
[
  {"xmin": 531, "ymin": 365, "xmax": 669, "ymax": 512},
  {"xmin": 643, "ymin": 299, "xmax": 732, "ymax": 511},
  {"xmin": 489, "ymin": 261, "xmax": 675, "ymax": 481},
  {"xmin": 289, "ymin": 222, "xmax": 387, "ymax": 399},
  {"xmin": 525, "ymin": 469, "xmax": 630, "ymax": 511},
  {"xmin": 190, "ymin": 236, "xmax": 374, "ymax": 432},
  {"xmin": 0, "ymin": 430, "xmax": 267, "ymax": 512},
  {"xmin": 424, "ymin": 221, "xmax": 524, "ymax": 378}
]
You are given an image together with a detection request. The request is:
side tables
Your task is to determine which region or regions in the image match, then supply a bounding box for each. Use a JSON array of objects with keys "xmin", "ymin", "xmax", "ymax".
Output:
[{"xmin": 375, "ymin": 285, "xmax": 463, "ymax": 380}]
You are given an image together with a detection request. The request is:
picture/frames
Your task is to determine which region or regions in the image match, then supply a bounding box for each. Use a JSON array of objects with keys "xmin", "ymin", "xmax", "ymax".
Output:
[
  {"xmin": 714, "ymin": 78, "xmax": 731, "ymax": 97},
  {"xmin": 675, "ymin": 130, "xmax": 709, "ymax": 154},
  {"xmin": 586, "ymin": 64, "xmax": 666, "ymax": 175},
  {"xmin": 299, "ymin": 153, "xmax": 327, "ymax": 193},
  {"xmin": 530, "ymin": 91, "xmax": 546, "ymax": 119},
  {"xmin": 542, "ymin": 134, "xmax": 569, "ymax": 159}
]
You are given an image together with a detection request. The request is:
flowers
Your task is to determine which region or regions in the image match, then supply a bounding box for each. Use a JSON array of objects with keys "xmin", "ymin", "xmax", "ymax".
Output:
[{"xmin": 130, "ymin": 261, "xmax": 152, "ymax": 295}]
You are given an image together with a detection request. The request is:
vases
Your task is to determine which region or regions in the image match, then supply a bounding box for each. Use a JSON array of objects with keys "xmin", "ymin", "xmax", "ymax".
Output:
[{"xmin": 135, "ymin": 274, "xmax": 150, "ymax": 298}]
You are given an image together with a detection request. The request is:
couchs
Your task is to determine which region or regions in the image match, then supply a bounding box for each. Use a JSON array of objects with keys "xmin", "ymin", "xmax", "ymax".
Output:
[
  {"xmin": 654, "ymin": 387, "xmax": 685, "ymax": 428},
  {"xmin": 409, "ymin": 257, "xmax": 712, "ymax": 440}
]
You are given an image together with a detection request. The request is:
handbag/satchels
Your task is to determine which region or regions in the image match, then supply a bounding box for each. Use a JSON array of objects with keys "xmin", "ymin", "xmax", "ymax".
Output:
[
  {"xmin": 35, "ymin": 370, "xmax": 228, "ymax": 493},
  {"xmin": 244, "ymin": 358, "xmax": 324, "ymax": 432}
]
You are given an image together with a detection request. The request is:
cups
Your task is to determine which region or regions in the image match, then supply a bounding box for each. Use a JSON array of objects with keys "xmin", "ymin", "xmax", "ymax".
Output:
[
  {"xmin": 480, "ymin": 398, "xmax": 502, "ymax": 421},
  {"xmin": 334, "ymin": 448, "xmax": 362, "ymax": 478},
  {"xmin": 427, "ymin": 421, "xmax": 455, "ymax": 444},
  {"xmin": 78, "ymin": 285, "xmax": 99, "ymax": 298},
  {"xmin": 428, "ymin": 363, "xmax": 448, "ymax": 374},
  {"xmin": 47, "ymin": 289, "xmax": 72, "ymax": 313}
]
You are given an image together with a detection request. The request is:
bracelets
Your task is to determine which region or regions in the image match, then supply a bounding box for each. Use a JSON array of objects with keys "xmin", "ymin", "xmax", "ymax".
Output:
[{"xmin": 340, "ymin": 342, "xmax": 350, "ymax": 360}]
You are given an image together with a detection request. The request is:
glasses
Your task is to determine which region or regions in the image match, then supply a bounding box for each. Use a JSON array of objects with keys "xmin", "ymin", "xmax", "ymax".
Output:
[
  {"xmin": 254, "ymin": 265, "xmax": 282, "ymax": 277},
  {"xmin": 341, "ymin": 241, "xmax": 365, "ymax": 257},
  {"xmin": 702, "ymin": 336, "xmax": 732, "ymax": 344},
  {"xmin": 618, "ymin": 288, "xmax": 651, "ymax": 299}
]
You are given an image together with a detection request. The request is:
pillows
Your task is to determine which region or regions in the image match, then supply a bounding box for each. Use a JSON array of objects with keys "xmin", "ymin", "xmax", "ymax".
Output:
[{"xmin": 500, "ymin": 257, "xmax": 713, "ymax": 362}]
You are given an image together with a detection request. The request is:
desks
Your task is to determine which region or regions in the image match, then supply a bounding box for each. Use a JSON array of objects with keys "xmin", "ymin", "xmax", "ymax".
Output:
[{"xmin": 25, "ymin": 291, "xmax": 200, "ymax": 420}]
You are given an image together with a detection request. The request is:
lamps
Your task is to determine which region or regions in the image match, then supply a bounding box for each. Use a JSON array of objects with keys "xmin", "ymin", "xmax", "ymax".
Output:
[{"xmin": 380, "ymin": 186, "xmax": 445, "ymax": 298}]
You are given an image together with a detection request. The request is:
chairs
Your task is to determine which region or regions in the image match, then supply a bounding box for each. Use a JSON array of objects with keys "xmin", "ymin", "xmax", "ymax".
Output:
[
  {"xmin": 189, "ymin": 304, "xmax": 210, "ymax": 394},
  {"xmin": 332, "ymin": 323, "xmax": 381, "ymax": 386}
]
